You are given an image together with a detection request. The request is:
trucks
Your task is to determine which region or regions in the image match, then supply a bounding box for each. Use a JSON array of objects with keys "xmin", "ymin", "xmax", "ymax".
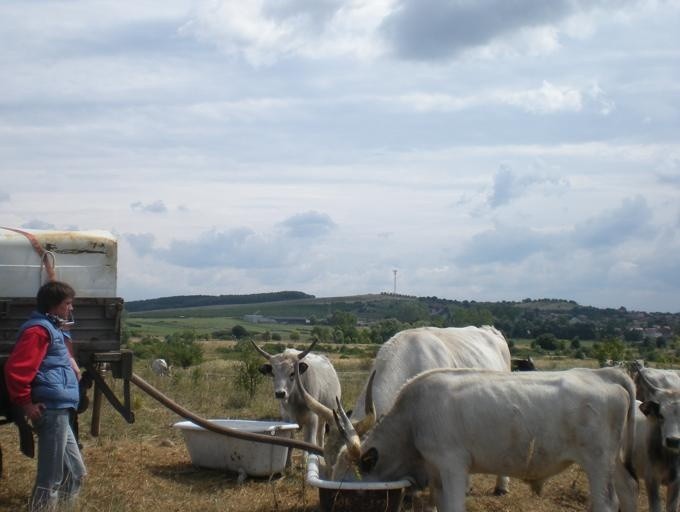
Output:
[{"xmin": 0, "ymin": 226, "xmax": 134, "ymax": 477}]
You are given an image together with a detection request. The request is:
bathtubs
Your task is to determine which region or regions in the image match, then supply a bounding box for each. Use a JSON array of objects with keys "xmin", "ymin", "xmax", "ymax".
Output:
[
  {"xmin": 305, "ymin": 448, "xmax": 413, "ymax": 512},
  {"xmin": 175, "ymin": 420, "xmax": 300, "ymax": 476}
]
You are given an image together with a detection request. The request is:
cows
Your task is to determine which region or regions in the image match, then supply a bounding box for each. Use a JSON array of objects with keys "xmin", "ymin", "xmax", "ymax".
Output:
[
  {"xmin": 326, "ymin": 364, "xmax": 641, "ymax": 511},
  {"xmin": 623, "ymin": 358, "xmax": 680, "ymax": 512},
  {"xmin": 151, "ymin": 358, "xmax": 169, "ymax": 377},
  {"xmin": 291, "ymin": 324, "xmax": 514, "ymax": 497},
  {"xmin": 247, "ymin": 336, "xmax": 342, "ymax": 452}
]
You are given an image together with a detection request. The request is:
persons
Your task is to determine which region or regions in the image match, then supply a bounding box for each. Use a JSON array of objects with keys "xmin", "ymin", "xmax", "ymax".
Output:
[{"xmin": 3, "ymin": 280, "xmax": 88, "ymax": 511}]
